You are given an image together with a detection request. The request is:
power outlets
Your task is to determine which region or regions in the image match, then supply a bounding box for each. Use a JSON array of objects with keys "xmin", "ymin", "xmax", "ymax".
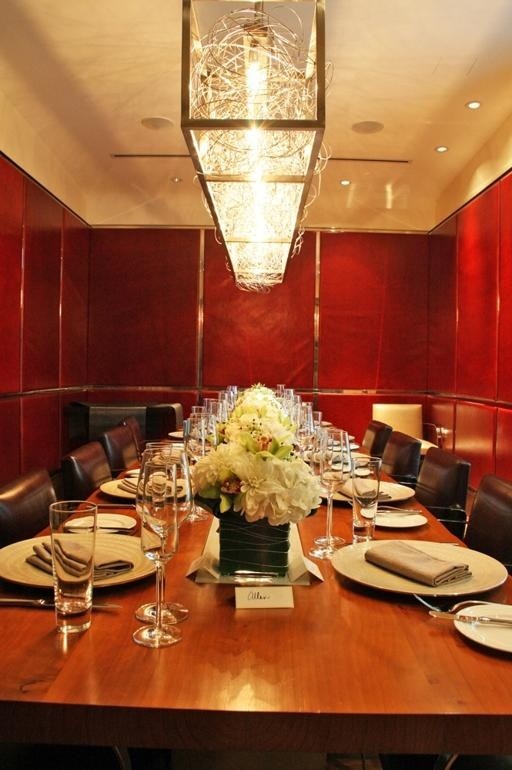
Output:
[{"xmin": 439, "ymin": 427, "xmax": 448, "ymax": 439}]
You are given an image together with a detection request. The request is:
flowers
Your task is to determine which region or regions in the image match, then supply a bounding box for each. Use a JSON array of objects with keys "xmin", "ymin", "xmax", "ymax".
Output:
[{"xmin": 189, "ymin": 382, "xmax": 322, "ymax": 526}]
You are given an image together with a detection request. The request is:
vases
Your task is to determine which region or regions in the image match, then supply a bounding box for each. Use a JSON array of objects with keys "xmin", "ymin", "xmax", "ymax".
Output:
[{"xmin": 219, "ymin": 513, "xmax": 291, "ymax": 579}]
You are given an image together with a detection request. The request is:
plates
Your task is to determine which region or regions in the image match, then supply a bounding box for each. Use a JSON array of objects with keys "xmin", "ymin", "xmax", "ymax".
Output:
[
  {"xmin": 374, "ymin": 511, "xmax": 428, "ymax": 529},
  {"xmin": 330, "ymin": 539, "xmax": 507, "ymax": 599},
  {"xmin": 453, "ymin": 605, "xmax": 510, "ymax": 655},
  {"xmin": 320, "ymin": 423, "xmax": 416, "ymax": 504},
  {"xmin": 0, "ymin": 431, "xmax": 184, "ymax": 589}
]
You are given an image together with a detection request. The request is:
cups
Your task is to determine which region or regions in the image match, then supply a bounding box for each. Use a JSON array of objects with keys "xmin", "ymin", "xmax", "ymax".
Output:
[{"xmin": 49, "ymin": 500, "xmax": 97, "ymax": 634}]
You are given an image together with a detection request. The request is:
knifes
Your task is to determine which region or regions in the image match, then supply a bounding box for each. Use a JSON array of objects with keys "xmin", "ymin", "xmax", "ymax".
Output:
[{"xmin": 0, "ymin": 598, "xmax": 123, "ymax": 608}]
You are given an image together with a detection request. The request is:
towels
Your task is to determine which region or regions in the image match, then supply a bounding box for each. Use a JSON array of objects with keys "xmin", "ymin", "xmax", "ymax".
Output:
[
  {"xmin": 25, "ymin": 539, "xmax": 134, "ymax": 581},
  {"xmin": 117, "ymin": 478, "xmax": 139, "ymax": 494},
  {"xmin": 365, "ymin": 539, "xmax": 472, "ymax": 587},
  {"xmin": 338, "ymin": 480, "xmax": 392, "ymax": 502}
]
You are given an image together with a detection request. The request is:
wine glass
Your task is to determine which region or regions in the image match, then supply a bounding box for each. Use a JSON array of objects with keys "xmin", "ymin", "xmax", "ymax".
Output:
[
  {"xmin": 272, "ymin": 382, "xmax": 353, "ymax": 561},
  {"xmin": 133, "ymin": 385, "xmax": 238, "ymax": 648}
]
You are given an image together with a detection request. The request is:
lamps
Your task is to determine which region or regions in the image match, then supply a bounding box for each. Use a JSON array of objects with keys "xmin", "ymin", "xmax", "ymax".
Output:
[
  {"xmin": 372, "ymin": 403, "xmax": 439, "ymax": 457},
  {"xmin": 180, "ymin": 0, "xmax": 327, "ymax": 287}
]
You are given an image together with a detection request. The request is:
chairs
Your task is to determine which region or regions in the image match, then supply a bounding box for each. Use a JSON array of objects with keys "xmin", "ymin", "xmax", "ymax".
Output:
[
  {"xmin": 1, "ymin": 467, "xmax": 57, "ymax": 548},
  {"xmin": 362, "ymin": 419, "xmax": 393, "ymax": 457},
  {"xmin": 119, "ymin": 415, "xmax": 146, "ymax": 457},
  {"xmin": 97, "ymin": 426, "xmax": 139, "ymax": 479},
  {"xmin": 63, "ymin": 441, "xmax": 112, "ymax": 500},
  {"xmin": 438, "ymin": 474, "xmax": 512, "ymax": 573},
  {"xmin": 381, "ymin": 430, "xmax": 422, "ymax": 480},
  {"xmin": 414, "ymin": 447, "xmax": 472, "ymax": 535}
]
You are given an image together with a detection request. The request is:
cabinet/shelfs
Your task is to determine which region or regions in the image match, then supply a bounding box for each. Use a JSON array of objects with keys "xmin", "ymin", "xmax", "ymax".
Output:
[{"xmin": 72, "ymin": 400, "xmax": 148, "ymax": 441}]
